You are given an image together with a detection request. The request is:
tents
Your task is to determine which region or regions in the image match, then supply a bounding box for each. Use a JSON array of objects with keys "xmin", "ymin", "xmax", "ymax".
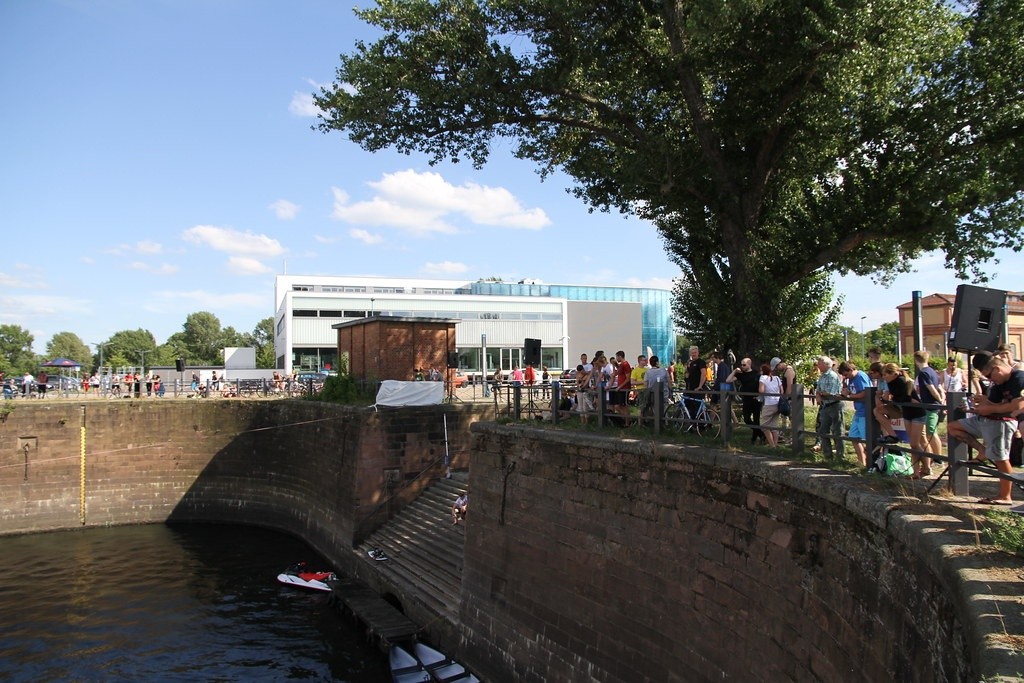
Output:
[{"xmin": 39, "ymin": 358, "xmax": 84, "ymax": 396}]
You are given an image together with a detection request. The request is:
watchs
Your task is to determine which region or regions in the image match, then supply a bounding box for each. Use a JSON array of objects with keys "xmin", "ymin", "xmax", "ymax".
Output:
[
  {"xmin": 847, "ymin": 393, "xmax": 852, "ymax": 399},
  {"xmin": 821, "ymin": 391, "xmax": 825, "ymax": 396}
]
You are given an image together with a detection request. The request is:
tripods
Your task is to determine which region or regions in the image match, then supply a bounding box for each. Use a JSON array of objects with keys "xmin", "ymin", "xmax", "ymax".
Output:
[
  {"xmin": 923, "ymin": 354, "xmax": 998, "ymax": 494},
  {"xmin": 520, "ymin": 365, "xmax": 542, "ymax": 412},
  {"xmin": 445, "ymin": 368, "xmax": 464, "ymax": 403}
]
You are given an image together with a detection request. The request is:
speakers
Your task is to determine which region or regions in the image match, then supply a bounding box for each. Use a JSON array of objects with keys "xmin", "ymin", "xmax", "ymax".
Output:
[
  {"xmin": 175, "ymin": 360, "xmax": 184, "ymax": 371},
  {"xmin": 947, "ymin": 284, "xmax": 1007, "ymax": 355},
  {"xmin": 524, "ymin": 338, "xmax": 541, "ymax": 365},
  {"xmin": 447, "ymin": 352, "xmax": 458, "ymax": 368}
]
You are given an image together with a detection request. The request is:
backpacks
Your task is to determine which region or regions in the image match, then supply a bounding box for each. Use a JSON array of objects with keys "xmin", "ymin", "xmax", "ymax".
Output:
[{"xmin": 195, "ymin": 376, "xmax": 200, "ymax": 384}]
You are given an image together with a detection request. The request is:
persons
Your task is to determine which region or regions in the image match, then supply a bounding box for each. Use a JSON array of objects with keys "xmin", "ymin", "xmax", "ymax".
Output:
[
  {"xmin": 413, "ymin": 367, "xmax": 442, "ymax": 382},
  {"xmin": 492, "ymin": 364, "xmax": 551, "ymax": 402},
  {"xmin": 0, "ymin": 371, "xmax": 162, "ymax": 400},
  {"xmin": 558, "ymin": 346, "xmax": 797, "ymax": 450},
  {"xmin": 268, "ymin": 363, "xmax": 331, "ymax": 397},
  {"xmin": 191, "ymin": 371, "xmax": 238, "ymax": 399},
  {"xmin": 810, "ymin": 346, "xmax": 1024, "ymax": 513},
  {"xmin": 452, "ymin": 491, "xmax": 468, "ymax": 526}
]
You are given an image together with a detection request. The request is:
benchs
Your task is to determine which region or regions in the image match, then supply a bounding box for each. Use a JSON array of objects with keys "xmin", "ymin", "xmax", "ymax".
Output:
[{"xmin": 391, "ymin": 659, "xmax": 470, "ymax": 683}]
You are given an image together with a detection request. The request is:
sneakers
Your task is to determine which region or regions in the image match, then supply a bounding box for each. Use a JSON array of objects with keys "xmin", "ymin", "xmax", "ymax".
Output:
[
  {"xmin": 1013, "ymin": 506, "xmax": 1024, "ymax": 513},
  {"xmin": 879, "ymin": 434, "xmax": 900, "ymax": 443},
  {"xmin": 371, "ymin": 547, "xmax": 384, "ymax": 558}
]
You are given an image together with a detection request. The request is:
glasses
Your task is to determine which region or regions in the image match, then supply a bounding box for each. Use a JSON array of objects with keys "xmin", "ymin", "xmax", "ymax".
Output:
[
  {"xmin": 867, "ymin": 353, "xmax": 872, "ymax": 358},
  {"xmin": 741, "ymin": 364, "xmax": 746, "ymax": 366},
  {"xmin": 869, "ymin": 370, "xmax": 877, "ymax": 375},
  {"xmin": 985, "ymin": 367, "xmax": 994, "ymax": 379}
]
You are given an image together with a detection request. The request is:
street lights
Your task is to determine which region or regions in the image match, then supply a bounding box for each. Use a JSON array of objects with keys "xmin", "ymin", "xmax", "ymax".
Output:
[
  {"xmin": 371, "ymin": 297, "xmax": 375, "ymax": 316},
  {"xmin": 861, "ymin": 317, "xmax": 866, "ymax": 363},
  {"xmin": 91, "ymin": 341, "xmax": 114, "ymax": 395},
  {"xmin": 135, "ymin": 350, "xmax": 152, "ymax": 366}
]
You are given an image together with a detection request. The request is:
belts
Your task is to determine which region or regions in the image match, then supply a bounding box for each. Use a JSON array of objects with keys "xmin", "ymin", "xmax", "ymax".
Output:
[{"xmin": 821, "ymin": 401, "xmax": 840, "ymax": 408}]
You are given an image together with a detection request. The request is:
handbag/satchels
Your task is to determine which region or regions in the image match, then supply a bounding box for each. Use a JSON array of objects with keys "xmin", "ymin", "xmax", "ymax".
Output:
[{"xmin": 778, "ymin": 396, "xmax": 791, "ymax": 416}]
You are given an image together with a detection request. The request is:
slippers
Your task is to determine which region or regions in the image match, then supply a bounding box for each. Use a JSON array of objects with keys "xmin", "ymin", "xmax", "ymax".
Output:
[
  {"xmin": 978, "ymin": 498, "xmax": 1012, "ymax": 505},
  {"xmin": 960, "ymin": 458, "xmax": 998, "ymax": 469}
]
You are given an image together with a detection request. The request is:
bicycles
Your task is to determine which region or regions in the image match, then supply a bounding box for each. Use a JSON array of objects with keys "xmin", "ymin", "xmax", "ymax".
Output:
[
  {"xmin": 662, "ymin": 387, "xmax": 721, "ymax": 440},
  {"xmin": 12, "ymin": 383, "xmax": 123, "ymax": 399},
  {"xmin": 239, "ymin": 381, "xmax": 270, "ymax": 398}
]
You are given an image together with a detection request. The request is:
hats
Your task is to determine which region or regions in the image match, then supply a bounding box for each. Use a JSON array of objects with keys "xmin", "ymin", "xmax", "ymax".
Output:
[{"xmin": 770, "ymin": 357, "xmax": 781, "ymax": 372}]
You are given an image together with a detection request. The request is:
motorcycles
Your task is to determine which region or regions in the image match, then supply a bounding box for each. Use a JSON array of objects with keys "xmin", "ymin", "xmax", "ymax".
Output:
[{"xmin": 277, "ymin": 562, "xmax": 338, "ymax": 592}]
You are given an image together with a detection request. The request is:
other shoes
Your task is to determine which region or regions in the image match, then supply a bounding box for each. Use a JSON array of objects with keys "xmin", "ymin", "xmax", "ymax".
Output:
[
  {"xmin": 919, "ymin": 465, "xmax": 930, "ymax": 475},
  {"xmin": 759, "ymin": 435, "xmax": 766, "ymax": 445},
  {"xmin": 778, "ymin": 436, "xmax": 785, "ymax": 443},
  {"xmin": 930, "ymin": 458, "xmax": 944, "ymax": 466},
  {"xmin": 812, "ymin": 445, "xmax": 822, "ymax": 451},
  {"xmin": 907, "ymin": 474, "xmax": 920, "ymax": 479}
]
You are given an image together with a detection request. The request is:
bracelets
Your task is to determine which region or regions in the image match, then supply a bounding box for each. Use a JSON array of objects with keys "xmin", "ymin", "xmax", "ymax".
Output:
[{"xmin": 618, "ymin": 387, "xmax": 620, "ymax": 389}]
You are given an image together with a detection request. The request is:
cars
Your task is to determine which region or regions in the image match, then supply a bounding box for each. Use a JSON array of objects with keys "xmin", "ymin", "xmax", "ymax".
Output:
[
  {"xmin": 560, "ymin": 368, "xmax": 576, "ymax": 383},
  {"xmin": 5, "ymin": 377, "xmax": 38, "ymax": 393},
  {"xmin": 294, "ymin": 372, "xmax": 327, "ymax": 383},
  {"xmin": 46, "ymin": 375, "xmax": 81, "ymax": 390},
  {"xmin": 456, "ymin": 371, "xmax": 469, "ymax": 388}
]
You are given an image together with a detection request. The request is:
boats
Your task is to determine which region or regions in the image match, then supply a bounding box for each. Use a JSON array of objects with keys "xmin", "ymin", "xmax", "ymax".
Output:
[
  {"xmin": 411, "ymin": 639, "xmax": 482, "ymax": 683},
  {"xmin": 388, "ymin": 642, "xmax": 436, "ymax": 683}
]
